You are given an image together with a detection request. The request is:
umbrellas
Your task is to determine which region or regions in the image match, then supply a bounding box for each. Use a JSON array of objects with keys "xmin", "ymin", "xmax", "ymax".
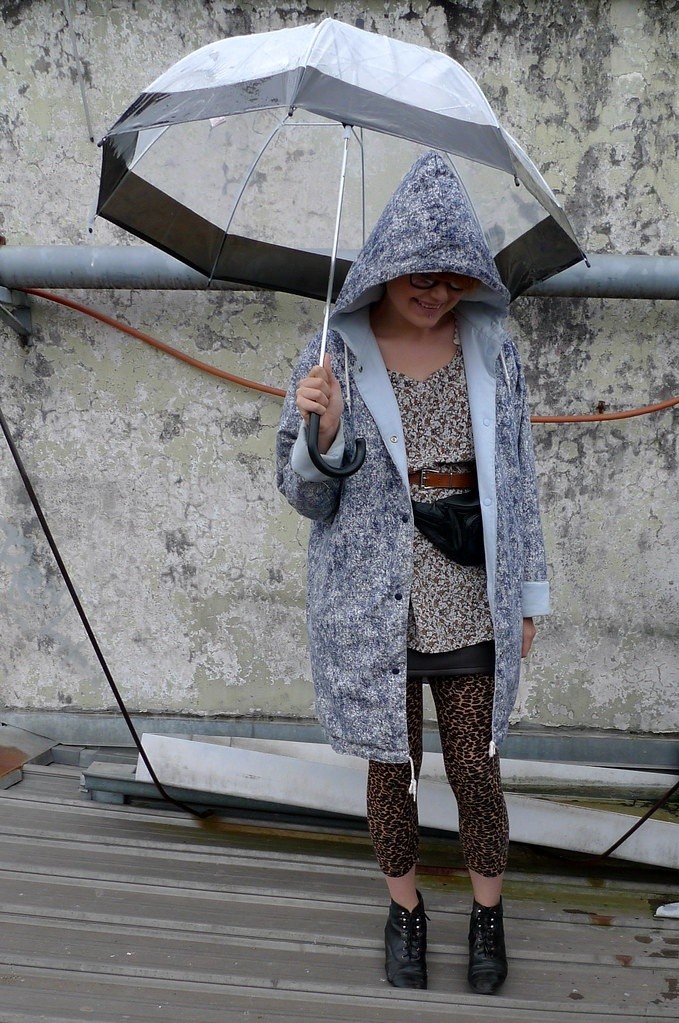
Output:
[{"xmin": 94, "ymin": 17, "xmax": 591, "ymax": 479}]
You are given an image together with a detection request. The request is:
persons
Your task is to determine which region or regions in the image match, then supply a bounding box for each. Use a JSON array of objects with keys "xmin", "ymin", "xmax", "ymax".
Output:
[{"xmin": 275, "ymin": 149, "xmax": 552, "ymax": 995}]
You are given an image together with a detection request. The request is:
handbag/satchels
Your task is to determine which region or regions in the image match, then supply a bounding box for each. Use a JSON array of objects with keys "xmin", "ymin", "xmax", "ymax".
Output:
[{"xmin": 411, "ymin": 490, "xmax": 487, "ymax": 568}]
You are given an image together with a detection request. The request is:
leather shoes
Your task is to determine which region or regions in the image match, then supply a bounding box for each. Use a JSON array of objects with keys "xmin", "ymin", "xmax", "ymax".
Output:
[
  {"xmin": 468, "ymin": 897, "xmax": 508, "ymax": 994},
  {"xmin": 383, "ymin": 888, "xmax": 431, "ymax": 990}
]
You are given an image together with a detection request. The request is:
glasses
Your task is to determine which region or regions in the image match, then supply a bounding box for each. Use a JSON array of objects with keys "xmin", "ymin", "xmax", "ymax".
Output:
[{"xmin": 409, "ymin": 270, "xmax": 478, "ymax": 292}]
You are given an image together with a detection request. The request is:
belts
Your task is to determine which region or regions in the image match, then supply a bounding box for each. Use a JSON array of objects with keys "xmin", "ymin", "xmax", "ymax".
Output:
[{"xmin": 409, "ymin": 468, "xmax": 479, "ymax": 490}]
